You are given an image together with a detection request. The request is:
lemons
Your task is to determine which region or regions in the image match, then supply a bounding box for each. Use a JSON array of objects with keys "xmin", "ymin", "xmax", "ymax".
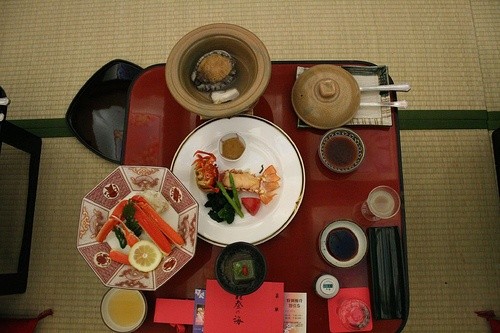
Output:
[{"xmin": 128, "ymin": 240, "xmax": 162, "ymax": 272}]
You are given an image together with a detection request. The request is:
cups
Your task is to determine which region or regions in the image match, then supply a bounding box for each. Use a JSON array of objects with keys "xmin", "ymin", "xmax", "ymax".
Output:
[{"xmin": 336, "ymin": 297, "xmax": 371, "ymax": 331}]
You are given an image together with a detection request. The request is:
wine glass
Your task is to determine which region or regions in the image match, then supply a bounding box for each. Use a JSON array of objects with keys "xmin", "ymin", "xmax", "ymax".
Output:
[{"xmin": 360, "ymin": 185, "xmax": 401, "ymax": 222}]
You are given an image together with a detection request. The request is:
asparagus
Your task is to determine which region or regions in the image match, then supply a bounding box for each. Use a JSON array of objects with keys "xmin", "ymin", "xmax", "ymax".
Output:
[{"xmin": 214, "ymin": 173, "xmax": 244, "ymax": 218}]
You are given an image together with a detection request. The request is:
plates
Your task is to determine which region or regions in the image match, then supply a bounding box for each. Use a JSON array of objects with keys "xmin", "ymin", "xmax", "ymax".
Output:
[
  {"xmin": 76, "ymin": 165, "xmax": 199, "ymax": 290},
  {"xmin": 317, "ymin": 218, "xmax": 368, "ymax": 269},
  {"xmin": 296, "ymin": 65, "xmax": 393, "ymax": 128},
  {"xmin": 318, "ymin": 128, "xmax": 366, "ymax": 174},
  {"xmin": 170, "ymin": 114, "xmax": 306, "ymax": 249}
]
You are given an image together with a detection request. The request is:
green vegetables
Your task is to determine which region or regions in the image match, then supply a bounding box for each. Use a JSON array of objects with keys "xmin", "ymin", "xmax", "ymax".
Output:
[
  {"xmin": 113, "ymin": 199, "xmax": 142, "ymax": 249},
  {"xmin": 205, "ymin": 189, "xmax": 234, "ymax": 224}
]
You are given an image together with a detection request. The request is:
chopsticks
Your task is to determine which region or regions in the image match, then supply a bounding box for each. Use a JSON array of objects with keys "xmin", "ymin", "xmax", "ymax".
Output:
[{"xmin": 367, "ymin": 227, "xmax": 402, "ymax": 321}]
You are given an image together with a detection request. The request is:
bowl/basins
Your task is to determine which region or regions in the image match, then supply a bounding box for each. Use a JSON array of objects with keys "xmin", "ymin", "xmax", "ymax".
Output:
[
  {"xmin": 164, "ymin": 22, "xmax": 272, "ymax": 119},
  {"xmin": 100, "ymin": 288, "xmax": 147, "ymax": 333},
  {"xmin": 214, "ymin": 241, "xmax": 267, "ymax": 296}
]
